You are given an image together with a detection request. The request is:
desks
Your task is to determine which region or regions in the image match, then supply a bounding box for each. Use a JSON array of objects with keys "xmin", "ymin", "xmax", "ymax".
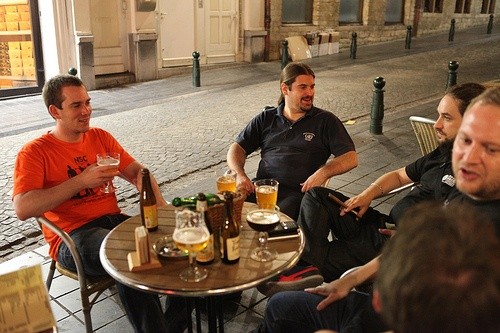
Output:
[{"xmin": 99, "ymin": 201, "xmax": 305, "ymax": 332}]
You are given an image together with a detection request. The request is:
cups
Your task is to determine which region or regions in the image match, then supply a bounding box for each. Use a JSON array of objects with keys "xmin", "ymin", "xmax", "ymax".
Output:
[
  {"xmin": 216, "ymin": 169, "xmax": 238, "ymax": 192},
  {"xmin": 254, "ymin": 178, "xmax": 278, "ymax": 211}
]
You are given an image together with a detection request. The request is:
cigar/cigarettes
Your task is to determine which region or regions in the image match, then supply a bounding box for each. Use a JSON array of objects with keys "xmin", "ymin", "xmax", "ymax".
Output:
[{"xmin": 328, "ymin": 193, "xmax": 358, "ymax": 215}]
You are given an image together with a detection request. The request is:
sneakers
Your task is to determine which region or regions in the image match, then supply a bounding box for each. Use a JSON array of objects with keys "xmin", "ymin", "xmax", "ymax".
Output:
[{"xmin": 256, "ymin": 266, "xmax": 323, "ymax": 298}]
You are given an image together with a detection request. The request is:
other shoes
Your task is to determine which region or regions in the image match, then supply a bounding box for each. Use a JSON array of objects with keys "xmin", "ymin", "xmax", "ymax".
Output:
[{"xmin": 204, "ymin": 290, "xmax": 242, "ymax": 301}]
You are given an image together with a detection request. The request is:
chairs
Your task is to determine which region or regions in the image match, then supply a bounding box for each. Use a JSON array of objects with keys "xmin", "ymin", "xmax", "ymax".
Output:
[
  {"xmin": 409, "ymin": 115, "xmax": 439, "ymax": 156},
  {"xmin": 37, "ymin": 216, "xmax": 115, "ymax": 333}
]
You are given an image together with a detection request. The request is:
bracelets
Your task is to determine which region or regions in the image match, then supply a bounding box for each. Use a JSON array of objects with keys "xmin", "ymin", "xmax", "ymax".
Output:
[{"xmin": 372, "ymin": 183, "xmax": 383, "ymax": 195}]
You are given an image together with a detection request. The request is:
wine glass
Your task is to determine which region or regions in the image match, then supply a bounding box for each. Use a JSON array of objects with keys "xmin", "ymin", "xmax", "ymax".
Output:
[
  {"xmin": 172, "ymin": 205, "xmax": 210, "ymax": 282},
  {"xmin": 96, "ymin": 153, "xmax": 121, "ymax": 194},
  {"xmin": 247, "ymin": 203, "xmax": 279, "ymax": 263}
]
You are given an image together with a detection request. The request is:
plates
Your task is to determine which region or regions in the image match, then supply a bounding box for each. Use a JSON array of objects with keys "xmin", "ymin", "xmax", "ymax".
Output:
[{"xmin": 152, "ymin": 234, "xmax": 189, "ymax": 260}]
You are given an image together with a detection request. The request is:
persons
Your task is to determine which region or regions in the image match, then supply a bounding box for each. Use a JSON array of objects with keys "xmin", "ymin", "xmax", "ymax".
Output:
[
  {"xmin": 11, "ymin": 74, "xmax": 191, "ymax": 333},
  {"xmin": 227, "ymin": 62, "xmax": 358, "ymax": 221},
  {"xmin": 261, "ymin": 79, "xmax": 500, "ymax": 333},
  {"xmin": 256, "ymin": 83, "xmax": 487, "ymax": 297}
]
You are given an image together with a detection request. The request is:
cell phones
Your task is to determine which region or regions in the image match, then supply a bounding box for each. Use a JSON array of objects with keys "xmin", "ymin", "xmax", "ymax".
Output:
[{"xmin": 268, "ymin": 221, "xmax": 299, "ymax": 236}]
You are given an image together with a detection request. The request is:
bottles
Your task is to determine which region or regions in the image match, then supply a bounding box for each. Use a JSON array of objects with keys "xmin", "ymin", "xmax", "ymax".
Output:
[
  {"xmin": 172, "ymin": 190, "xmax": 226, "ymax": 208},
  {"xmin": 139, "ymin": 168, "xmax": 159, "ymax": 233},
  {"xmin": 194, "ymin": 193, "xmax": 215, "ymax": 265},
  {"xmin": 219, "ymin": 193, "xmax": 241, "ymax": 264}
]
